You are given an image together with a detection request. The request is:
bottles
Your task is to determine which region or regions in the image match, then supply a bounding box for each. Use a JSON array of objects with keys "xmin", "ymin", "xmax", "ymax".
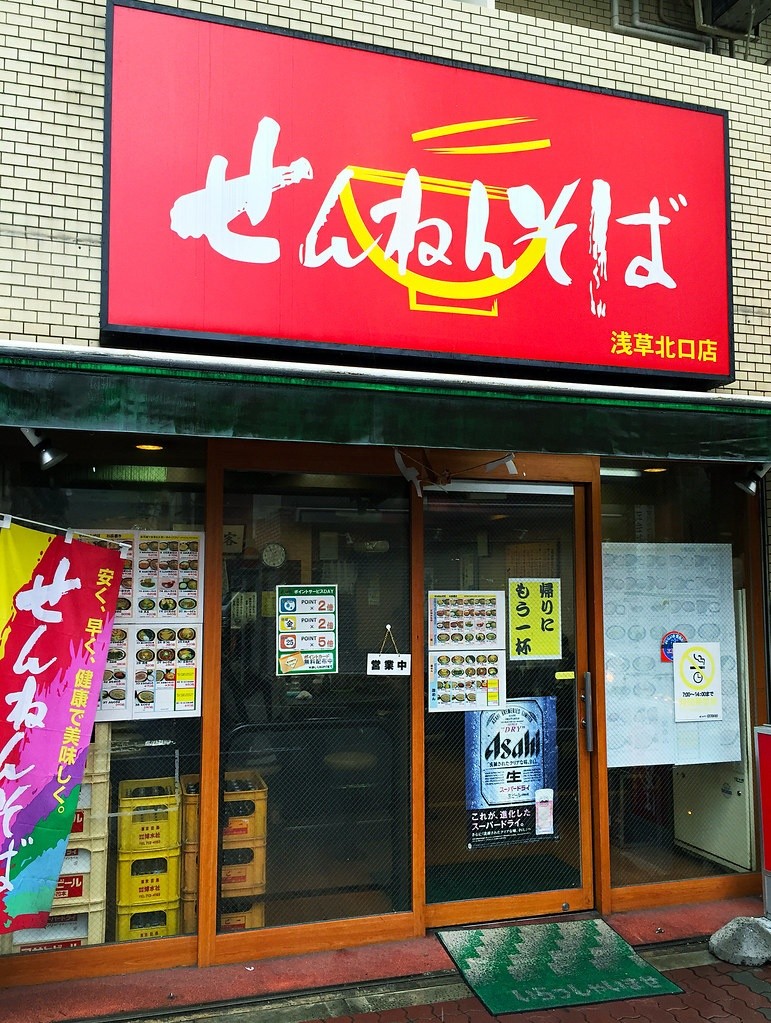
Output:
[{"xmin": 126, "ymin": 774, "xmax": 261, "ymax": 930}]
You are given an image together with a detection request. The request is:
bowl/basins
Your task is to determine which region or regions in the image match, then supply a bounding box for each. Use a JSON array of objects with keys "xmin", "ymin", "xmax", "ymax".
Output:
[
  {"xmin": 436, "ymin": 597, "xmax": 498, "ymax": 702},
  {"xmin": 91, "ymin": 536, "xmax": 198, "ymax": 705}
]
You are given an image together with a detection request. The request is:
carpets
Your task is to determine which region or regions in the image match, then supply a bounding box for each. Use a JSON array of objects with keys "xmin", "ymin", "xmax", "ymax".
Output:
[{"xmin": 433, "ymin": 915, "xmax": 685, "ymax": 1017}]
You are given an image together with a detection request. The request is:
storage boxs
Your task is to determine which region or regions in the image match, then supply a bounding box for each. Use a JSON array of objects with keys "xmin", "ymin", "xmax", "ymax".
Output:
[
  {"xmin": 69, "ymin": 772, "xmax": 109, "ymax": 840},
  {"xmin": 117, "ymin": 776, "xmax": 183, "ymax": 853},
  {"xmin": 180, "ymin": 771, "xmax": 268, "ymax": 844},
  {"xmin": 182, "ymin": 884, "xmax": 266, "ymax": 935},
  {"xmin": 49, "ymin": 836, "xmax": 107, "ymax": 906},
  {"xmin": 0, "ymin": 901, "xmax": 106, "ymax": 955},
  {"xmin": 82, "ymin": 722, "xmax": 110, "ymax": 773},
  {"xmin": 180, "ymin": 840, "xmax": 267, "ymax": 894},
  {"xmin": 114, "ymin": 901, "xmax": 182, "ymax": 939},
  {"xmin": 117, "ymin": 847, "xmax": 181, "ymax": 908}
]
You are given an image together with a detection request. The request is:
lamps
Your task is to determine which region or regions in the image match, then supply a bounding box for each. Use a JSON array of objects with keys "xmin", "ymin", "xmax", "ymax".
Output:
[
  {"xmin": 734, "ymin": 463, "xmax": 771, "ymax": 497},
  {"xmin": 20, "ymin": 427, "xmax": 68, "ymax": 471}
]
